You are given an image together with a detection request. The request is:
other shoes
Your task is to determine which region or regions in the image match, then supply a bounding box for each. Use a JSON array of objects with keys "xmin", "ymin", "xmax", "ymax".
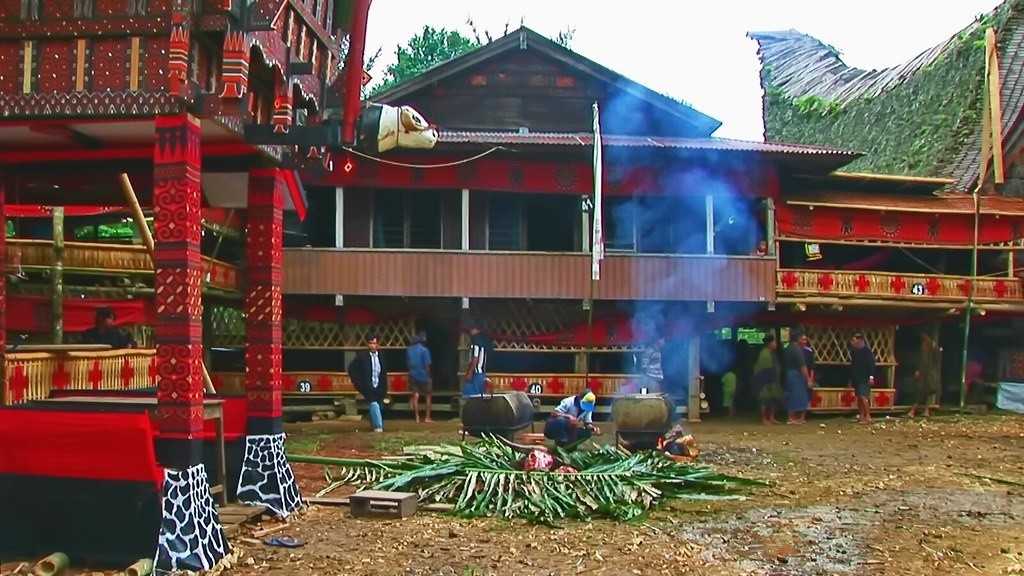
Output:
[{"xmin": 374, "ymin": 428, "xmax": 383, "ymax": 433}]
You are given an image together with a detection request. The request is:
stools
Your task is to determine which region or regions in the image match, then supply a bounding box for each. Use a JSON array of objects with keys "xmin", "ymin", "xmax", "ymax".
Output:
[{"xmin": 348, "ymin": 490, "xmax": 419, "ymax": 516}]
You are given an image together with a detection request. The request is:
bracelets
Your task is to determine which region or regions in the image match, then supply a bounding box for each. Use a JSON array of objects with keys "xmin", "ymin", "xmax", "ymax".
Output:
[{"xmin": 869, "ymin": 375, "xmax": 874, "ymax": 379}]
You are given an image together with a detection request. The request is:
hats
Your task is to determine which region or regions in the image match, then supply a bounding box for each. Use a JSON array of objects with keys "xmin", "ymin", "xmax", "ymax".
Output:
[
  {"xmin": 853, "ymin": 331, "xmax": 862, "ymax": 337},
  {"xmin": 580, "ymin": 389, "xmax": 596, "ymax": 411},
  {"xmin": 763, "ymin": 335, "xmax": 775, "ymax": 345}
]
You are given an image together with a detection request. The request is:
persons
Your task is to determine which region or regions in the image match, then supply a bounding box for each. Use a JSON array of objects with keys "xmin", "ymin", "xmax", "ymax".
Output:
[
  {"xmin": 406, "ymin": 330, "xmax": 437, "ymax": 423},
  {"xmin": 80, "ymin": 307, "xmax": 139, "ymax": 350},
  {"xmin": 900, "ymin": 327, "xmax": 943, "ymax": 419},
  {"xmin": 348, "ymin": 335, "xmax": 387, "ymax": 433},
  {"xmin": 637, "ymin": 327, "xmax": 815, "ymax": 426},
  {"xmin": 543, "ymin": 388, "xmax": 602, "ymax": 454},
  {"xmin": 849, "ymin": 332, "xmax": 876, "ymax": 425},
  {"xmin": 749, "ymin": 239, "xmax": 768, "ymax": 257},
  {"xmin": 458, "ymin": 321, "xmax": 491, "ymax": 435}
]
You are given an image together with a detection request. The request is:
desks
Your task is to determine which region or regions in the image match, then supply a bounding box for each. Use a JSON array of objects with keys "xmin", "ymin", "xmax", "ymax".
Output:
[{"xmin": 25, "ymin": 396, "xmax": 228, "ymax": 506}]
